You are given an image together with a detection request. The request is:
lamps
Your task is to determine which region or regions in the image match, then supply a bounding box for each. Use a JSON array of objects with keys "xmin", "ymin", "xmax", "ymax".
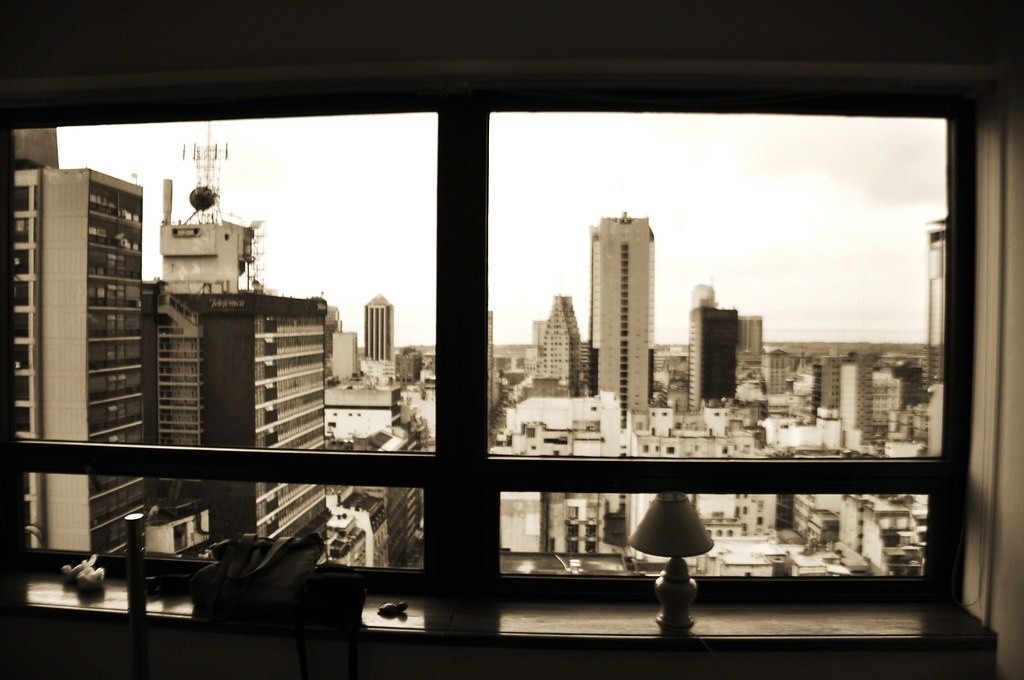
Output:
[{"xmin": 628, "ymin": 492, "xmax": 715, "ymax": 636}]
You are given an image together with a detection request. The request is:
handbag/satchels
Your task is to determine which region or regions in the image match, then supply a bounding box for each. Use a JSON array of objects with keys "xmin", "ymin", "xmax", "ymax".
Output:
[
  {"xmin": 188, "ymin": 532, "xmax": 323, "ymax": 621},
  {"xmin": 303, "ymin": 562, "xmax": 366, "ymax": 626}
]
